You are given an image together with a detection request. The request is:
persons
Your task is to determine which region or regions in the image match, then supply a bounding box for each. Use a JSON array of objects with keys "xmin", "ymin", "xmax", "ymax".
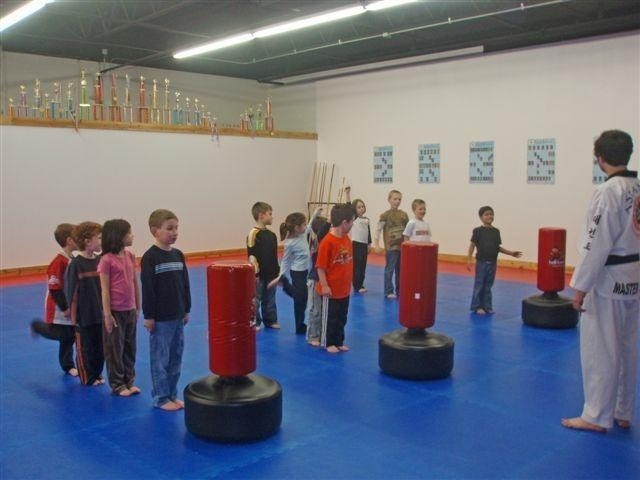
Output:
[
  {"xmin": 315, "ymin": 203, "xmax": 357, "ymax": 355},
  {"xmin": 301, "ymin": 214, "xmax": 331, "ymax": 348},
  {"xmin": 558, "ymin": 123, "xmax": 639, "ymax": 440},
  {"xmin": 141, "ymin": 207, "xmax": 192, "ymax": 412},
  {"xmin": 266, "ymin": 206, "xmax": 325, "ymax": 336},
  {"xmin": 93, "ymin": 217, "xmax": 143, "ymax": 397},
  {"xmin": 464, "ymin": 204, "xmax": 524, "ymax": 318},
  {"xmin": 245, "ymin": 202, "xmax": 285, "ymax": 331},
  {"xmin": 373, "ymin": 189, "xmax": 410, "ymax": 299},
  {"xmin": 343, "ymin": 183, "xmax": 373, "ymax": 296},
  {"xmin": 25, "ymin": 221, "xmax": 83, "ymax": 378},
  {"xmin": 65, "ymin": 221, "xmax": 108, "ymax": 387},
  {"xmin": 403, "ymin": 199, "xmax": 433, "ymax": 242}
]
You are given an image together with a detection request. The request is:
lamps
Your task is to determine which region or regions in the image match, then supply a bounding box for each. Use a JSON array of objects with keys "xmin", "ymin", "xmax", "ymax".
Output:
[{"xmin": 172, "ymin": 0, "xmax": 427, "ymax": 62}]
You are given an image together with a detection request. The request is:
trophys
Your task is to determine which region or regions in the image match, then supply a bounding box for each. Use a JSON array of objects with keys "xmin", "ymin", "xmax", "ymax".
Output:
[{"xmin": 6, "ymin": 70, "xmax": 276, "ymax": 138}]
